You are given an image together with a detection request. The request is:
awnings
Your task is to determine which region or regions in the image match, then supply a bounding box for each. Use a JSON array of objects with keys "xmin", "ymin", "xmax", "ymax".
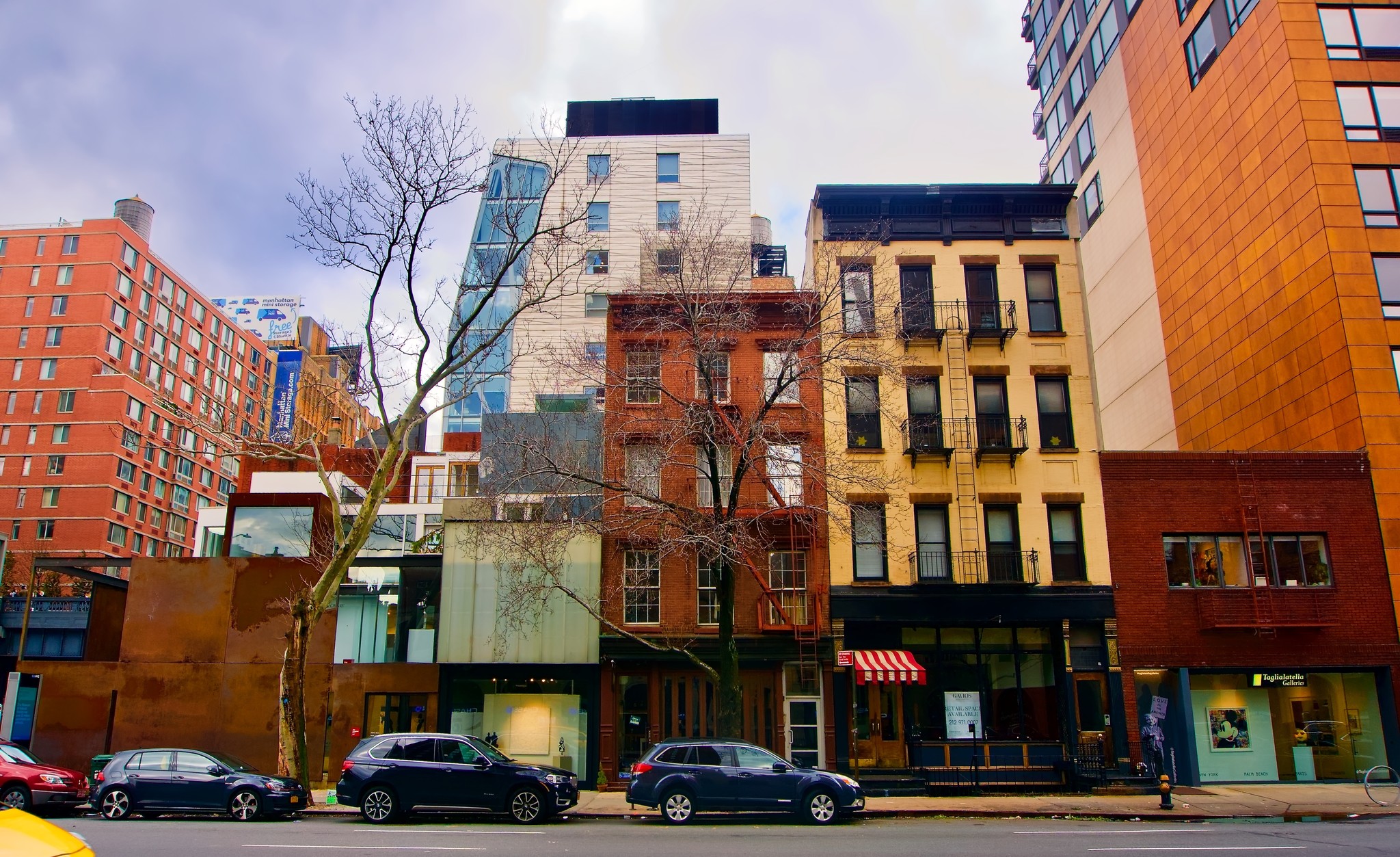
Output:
[{"xmin": 849, "ymin": 650, "xmax": 928, "ymax": 686}]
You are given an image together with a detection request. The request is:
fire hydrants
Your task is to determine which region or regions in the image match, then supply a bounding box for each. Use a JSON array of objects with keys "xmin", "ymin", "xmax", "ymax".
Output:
[{"xmin": 1159, "ymin": 774, "xmax": 1175, "ymax": 810}]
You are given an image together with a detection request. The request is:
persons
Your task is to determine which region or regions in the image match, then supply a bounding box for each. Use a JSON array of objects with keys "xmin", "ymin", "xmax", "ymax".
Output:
[{"xmin": 1141, "ymin": 712, "xmax": 1164, "ymax": 779}]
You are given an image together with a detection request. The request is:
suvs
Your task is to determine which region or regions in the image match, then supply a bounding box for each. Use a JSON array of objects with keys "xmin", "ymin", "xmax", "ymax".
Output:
[
  {"xmin": 0, "ymin": 736, "xmax": 92, "ymax": 816},
  {"xmin": 332, "ymin": 731, "xmax": 584, "ymax": 830}
]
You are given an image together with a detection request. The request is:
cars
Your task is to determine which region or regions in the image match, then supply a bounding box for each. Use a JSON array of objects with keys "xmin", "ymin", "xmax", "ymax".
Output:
[
  {"xmin": 625, "ymin": 736, "xmax": 868, "ymax": 827},
  {"xmin": 87, "ymin": 749, "xmax": 311, "ymax": 824}
]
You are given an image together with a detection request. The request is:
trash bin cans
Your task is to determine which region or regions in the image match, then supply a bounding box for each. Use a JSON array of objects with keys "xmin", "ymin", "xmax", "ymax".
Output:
[{"xmin": 90, "ymin": 754, "xmax": 114, "ymax": 788}]
